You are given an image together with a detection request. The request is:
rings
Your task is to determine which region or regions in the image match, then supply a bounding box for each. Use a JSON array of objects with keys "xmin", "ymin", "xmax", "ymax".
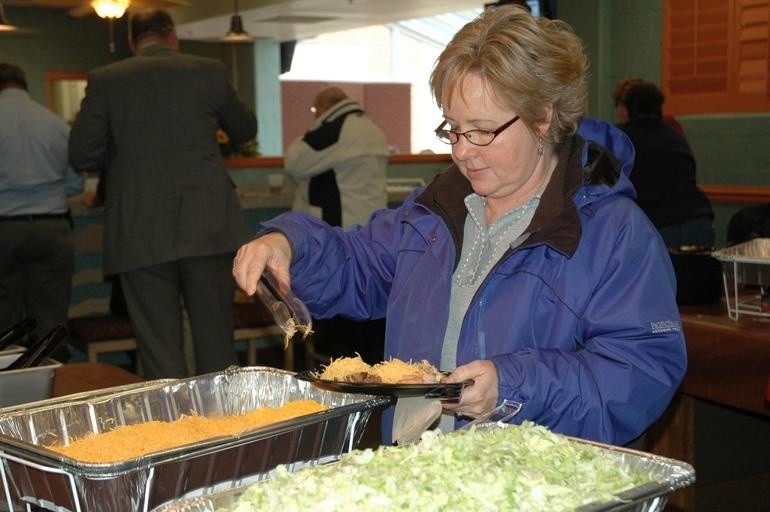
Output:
[{"xmin": 457, "ymin": 410, "xmax": 463, "ymax": 417}]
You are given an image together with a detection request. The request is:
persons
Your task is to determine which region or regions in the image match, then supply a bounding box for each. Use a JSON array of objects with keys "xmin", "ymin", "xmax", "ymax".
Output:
[
  {"xmin": 231, "ymin": 6, "xmax": 687, "ymax": 447},
  {"xmin": 69, "ymin": 8, "xmax": 258, "ymax": 379},
  {"xmin": 0, "ymin": 62, "xmax": 88, "ymax": 367},
  {"xmin": 284, "ymin": 88, "xmax": 388, "ymax": 365},
  {"xmin": 609, "ymin": 76, "xmax": 722, "ymax": 303}
]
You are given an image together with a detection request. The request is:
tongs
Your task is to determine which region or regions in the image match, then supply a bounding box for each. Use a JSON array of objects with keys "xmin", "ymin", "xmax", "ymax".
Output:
[{"xmin": 255, "ymin": 266, "xmax": 313, "ymax": 339}]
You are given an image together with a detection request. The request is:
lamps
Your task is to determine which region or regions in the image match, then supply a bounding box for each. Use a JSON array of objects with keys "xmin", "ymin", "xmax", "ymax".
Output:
[{"xmin": 223, "ymin": 0, "xmax": 253, "ymax": 44}]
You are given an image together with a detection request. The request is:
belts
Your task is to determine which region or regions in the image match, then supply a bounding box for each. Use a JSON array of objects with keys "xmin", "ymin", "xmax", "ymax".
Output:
[{"xmin": 1, "ymin": 213, "xmax": 68, "ymax": 222}]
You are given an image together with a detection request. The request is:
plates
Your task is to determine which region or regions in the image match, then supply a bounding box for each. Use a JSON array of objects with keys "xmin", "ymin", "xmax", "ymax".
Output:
[{"xmin": 293, "ymin": 368, "xmax": 476, "ymax": 400}]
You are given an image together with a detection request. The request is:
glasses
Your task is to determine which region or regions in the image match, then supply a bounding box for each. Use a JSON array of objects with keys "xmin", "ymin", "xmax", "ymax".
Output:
[{"xmin": 434, "ymin": 116, "xmax": 520, "ymax": 146}]
